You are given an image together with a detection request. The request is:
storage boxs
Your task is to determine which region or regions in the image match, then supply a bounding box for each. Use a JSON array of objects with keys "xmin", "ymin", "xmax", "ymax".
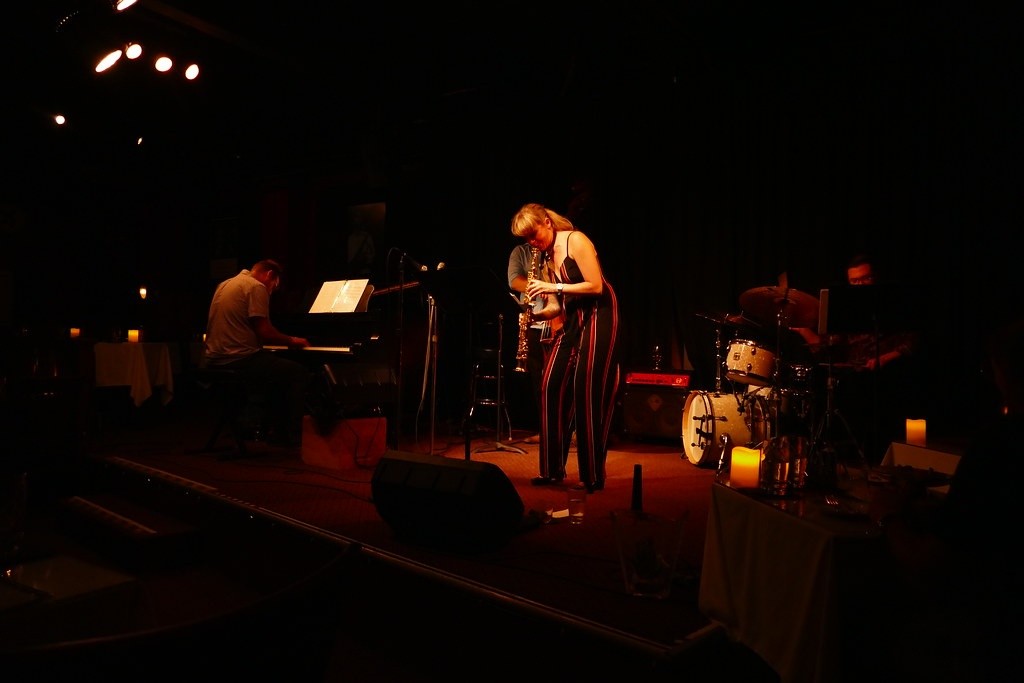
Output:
[{"xmin": 299, "ymin": 415, "xmax": 386, "ymax": 473}]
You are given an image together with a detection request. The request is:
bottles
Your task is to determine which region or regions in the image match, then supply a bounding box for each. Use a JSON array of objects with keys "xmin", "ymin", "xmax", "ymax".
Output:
[{"xmin": 577, "ymin": 482, "xmax": 589, "ymax": 517}]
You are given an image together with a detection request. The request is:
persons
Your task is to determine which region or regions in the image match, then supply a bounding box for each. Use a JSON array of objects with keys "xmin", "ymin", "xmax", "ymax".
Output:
[
  {"xmin": 507, "ymin": 244, "xmax": 577, "ymax": 448},
  {"xmin": 873, "ymin": 320, "xmax": 1024, "ymax": 596},
  {"xmin": 847, "ymin": 264, "xmax": 914, "ymax": 370},
  {"xmin": 206, "ymin": 259, "xmax": 313, "ymax": 449},
  {"xmin": 511, "ymin": 203, "xmax": 618, "ymax": 492}
]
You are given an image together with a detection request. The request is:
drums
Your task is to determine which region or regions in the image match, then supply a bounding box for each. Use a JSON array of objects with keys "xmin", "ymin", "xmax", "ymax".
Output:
[
  {"xmin": 723, "ymin": 339, "xmax": 780, "ymax": 389},
  {"xmin": 682, "ymin": 390, "xmax": 766, "ymax": 465}
]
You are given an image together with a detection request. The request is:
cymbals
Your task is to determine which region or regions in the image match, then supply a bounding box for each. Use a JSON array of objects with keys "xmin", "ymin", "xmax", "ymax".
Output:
[
  {"xmin": 740, "ymin": 286, "xmax": 819, "ymax": 328},
  {"xmin": 700, "ymin": 311, "xmax": 742, "ymax": 327},
  {"xmin": 816, "ymin": 363, "xmax": 867, "ymax": 367}
]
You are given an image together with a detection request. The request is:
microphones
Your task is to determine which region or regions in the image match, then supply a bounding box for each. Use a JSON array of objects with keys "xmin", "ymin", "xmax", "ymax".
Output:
[{"xmin": 395, "ymin": 248, "xmax": 435, "ymax": 282}]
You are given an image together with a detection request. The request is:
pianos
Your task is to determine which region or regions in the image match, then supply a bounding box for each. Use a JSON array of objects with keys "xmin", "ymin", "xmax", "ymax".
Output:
[{"xmin": 203, "ymin": 281, "xmax": 438, "ymax": 450}]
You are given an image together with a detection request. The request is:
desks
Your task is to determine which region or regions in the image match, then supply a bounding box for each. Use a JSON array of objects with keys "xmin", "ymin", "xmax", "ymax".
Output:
[
  {"xmin": 93, "ymin": 342, "xmax": 180, "ymax": 444},
  {"xmin": 893, "ymin": 435, "xmax": 970, "ymax": 526},
  {"xmin": 712, "ymin": 480, "xmax": 889, "ymax": 683}
]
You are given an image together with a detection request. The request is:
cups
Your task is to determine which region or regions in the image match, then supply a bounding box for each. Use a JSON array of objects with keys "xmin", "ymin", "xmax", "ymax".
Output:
[{"xmin": 567, "ymin": 487, "xmax": 586, "ymax": 525}]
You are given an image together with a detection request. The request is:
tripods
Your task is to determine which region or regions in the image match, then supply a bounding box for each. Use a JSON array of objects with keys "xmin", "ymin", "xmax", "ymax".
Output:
[
  {"xmin": 471, "ymin": 266, "xmax": 531, "ymax": 457},
  {"xmin": 802, "ymin": 353, "xmax": 870, "ymax": 490}
]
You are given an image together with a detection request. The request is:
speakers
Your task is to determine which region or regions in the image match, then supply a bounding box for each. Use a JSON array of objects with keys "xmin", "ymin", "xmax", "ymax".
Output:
[
  {"xmin": 370, "ymin": 449, "xmax": 524, "ymax": 555},
  {"xmin": 624, "ymin": 388, "xmax": 688, "ymax": 439}
]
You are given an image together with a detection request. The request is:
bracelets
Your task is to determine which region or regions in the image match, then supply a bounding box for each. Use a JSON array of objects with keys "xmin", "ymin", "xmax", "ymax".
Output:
[
  {"xmin": 876, "ymin": 515, "xmax": 894, "ymax": 528},
  {"xmin": 288, "ymin": 336, "xmax": 293, "ymax": 342},
  {"xmin": 557, "ymin": 283, "xmax": 563, "ymax": 290}
]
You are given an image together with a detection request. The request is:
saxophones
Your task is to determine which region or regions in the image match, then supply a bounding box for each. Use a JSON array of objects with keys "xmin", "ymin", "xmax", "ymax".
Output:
[{"xmin": 514, "ymin": 248, "xmax": 542, "ymax": 373}]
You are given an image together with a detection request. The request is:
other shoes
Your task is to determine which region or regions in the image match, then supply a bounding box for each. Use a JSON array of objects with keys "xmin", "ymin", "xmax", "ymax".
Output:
[
  {"xmin": 576, "ymin": 480, "xmax": 604, "ymax": 490},
  {"xmin": 533, "ymin": 472, "xmax": 563, "ymax": 487},
  {"xmin": 524, "ymin": 434, "xmax": 540, "ymax": 444}
]
